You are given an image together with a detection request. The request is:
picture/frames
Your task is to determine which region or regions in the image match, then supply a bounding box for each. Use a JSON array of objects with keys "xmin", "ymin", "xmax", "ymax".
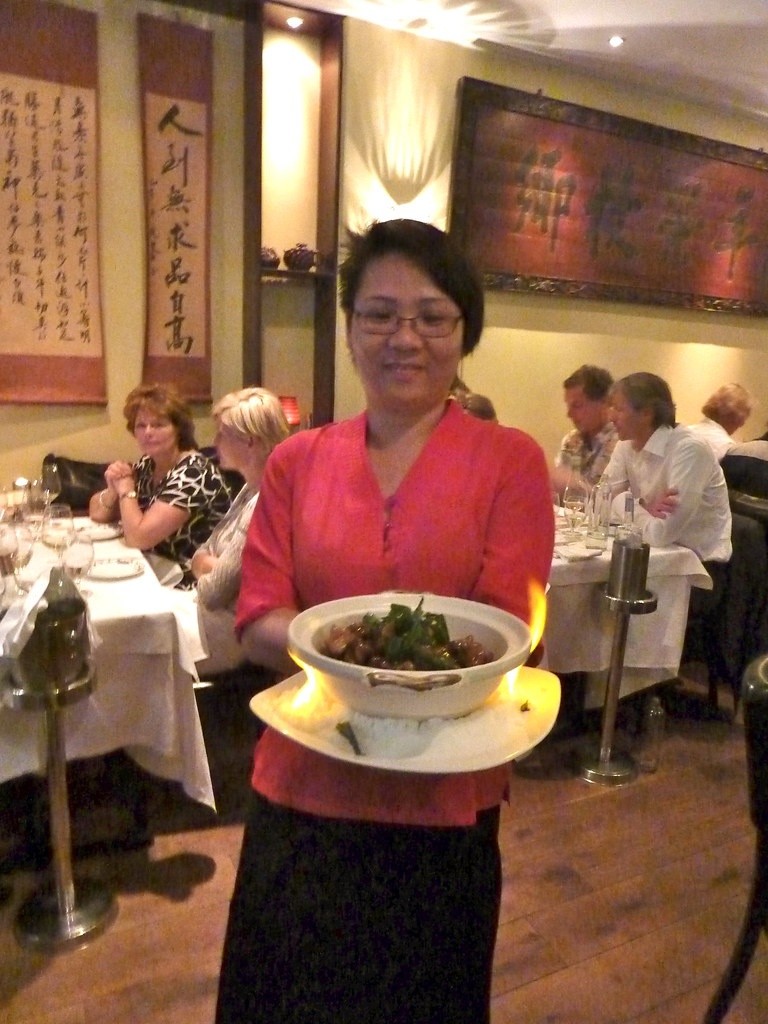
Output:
[{"xmin": 445, "ymin": 76, "xmax": 768, "ymax": 321}]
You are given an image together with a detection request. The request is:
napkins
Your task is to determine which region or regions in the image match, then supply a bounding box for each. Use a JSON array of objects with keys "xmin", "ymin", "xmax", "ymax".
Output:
[{"xmin": 553, "ymin": 544, "xmax": 602, "ymax": 563}]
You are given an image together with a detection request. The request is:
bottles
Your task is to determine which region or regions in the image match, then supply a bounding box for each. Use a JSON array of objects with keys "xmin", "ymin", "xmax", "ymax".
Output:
[
  {"xmin": 586, "ymin": 473, "xmax": 613, "ymax": 551},
  {"xmin": 637, "ymin": 697, "xmax": 665, "ymax": 773},
  {"xmin": 562, "ymin": 456, "xmax": 589, "ymax": 503}
]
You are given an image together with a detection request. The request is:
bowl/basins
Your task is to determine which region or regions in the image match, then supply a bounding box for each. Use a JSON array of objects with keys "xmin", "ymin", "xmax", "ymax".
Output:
[{"xmin": 286, "ymin": 593, "xmax": 532, "ymax": 723}]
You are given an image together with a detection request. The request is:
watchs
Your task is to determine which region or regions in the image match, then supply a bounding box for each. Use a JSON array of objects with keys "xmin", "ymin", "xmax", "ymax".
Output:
[{"xmin": 119, "ymin": 490, "xmax": 137, "ymax": 502}]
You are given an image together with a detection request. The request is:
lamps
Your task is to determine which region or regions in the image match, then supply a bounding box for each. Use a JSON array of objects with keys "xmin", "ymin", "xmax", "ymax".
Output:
[{"xmin": 279, "ymin": 395, "xmax": 301, "ymax": 424}]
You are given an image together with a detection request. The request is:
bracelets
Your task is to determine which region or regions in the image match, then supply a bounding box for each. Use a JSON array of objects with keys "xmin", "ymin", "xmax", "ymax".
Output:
[{"xmin": 100, "ymin": 488, "xmax": 119, "ymax": 512}]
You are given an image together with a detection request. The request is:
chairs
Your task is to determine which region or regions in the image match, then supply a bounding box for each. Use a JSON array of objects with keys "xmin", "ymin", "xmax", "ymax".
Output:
[
  {"xmin": 130, "ymin": 668, "xmax": 277, "ymax": 827},
  {"xmin": 632, "ymin": 454, "xmax": 768, "ymax": 1024}
]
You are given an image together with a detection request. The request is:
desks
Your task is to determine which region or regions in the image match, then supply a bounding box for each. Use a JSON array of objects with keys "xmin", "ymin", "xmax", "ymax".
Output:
[
  {"xmin": 540, "ymin": 505, "xmax": 715, "ymax": 744},
  {"xmin": 0, "ymin": 505, "xmax": 221, "ymax": 874}
]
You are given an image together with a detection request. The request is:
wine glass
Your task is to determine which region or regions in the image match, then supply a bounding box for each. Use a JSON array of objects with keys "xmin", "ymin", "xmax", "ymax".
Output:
[
  {"xmin": 0, "ymin": 463, "xmax": 93, "ymax": 610},
  {"xmin": 550, "ymin": 491, "xmax": 588, "ymax": 539}
]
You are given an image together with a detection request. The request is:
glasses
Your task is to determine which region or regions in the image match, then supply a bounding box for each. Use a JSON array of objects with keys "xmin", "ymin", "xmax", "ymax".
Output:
[{"xmin": 350, "ymin": 306, "xmax": 463, "ymax": 339}]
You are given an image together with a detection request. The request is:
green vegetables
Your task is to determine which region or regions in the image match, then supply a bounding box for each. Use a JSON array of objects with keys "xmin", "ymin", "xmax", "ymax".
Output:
[{"xmin": 362, "ymin": 596, "xmax": 464, "ymax": 671}]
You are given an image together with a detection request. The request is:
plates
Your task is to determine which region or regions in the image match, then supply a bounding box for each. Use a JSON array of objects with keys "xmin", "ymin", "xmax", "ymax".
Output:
[
  {"xmin": 249, "ymin": 665, "xmax": 562, "ymax": 775},
  {"xmin": 76, "ymin": 523, "xmax": 123, "ymax": 541},
  {"xmin": 85, "ymin": 559, "xmax": 146, "ymax": 580}
]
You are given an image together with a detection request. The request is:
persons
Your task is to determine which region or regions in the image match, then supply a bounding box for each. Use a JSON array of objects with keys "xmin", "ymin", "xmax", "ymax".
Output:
[
  {"xmin": 191, "ymin": 387, "xmax": 295, "ymax": 612},
  {"xmin": 214, "ymin": 219, "xmax": 555, "ymax": 1024},
  {"xmin": 590, "ymin": 372, "xmax": 733, "ymax": 620},
  {"xmin": 728, "ymin": 430, "xmax": 768, "ymax": 461},
  {"xmin": 552, "ymin": 365, "xmax": 619, "ymax": 515},
  {"xmin": 686, "ymin": 383, "xmax": 752, "ymax": 461},
  {"xmin": 457, "ymin": 392, "xmax": 499, "ymax": 424},
  {"xmin": 90, "ymin": 382, "xmax": 233, "ymax": 591}
]
